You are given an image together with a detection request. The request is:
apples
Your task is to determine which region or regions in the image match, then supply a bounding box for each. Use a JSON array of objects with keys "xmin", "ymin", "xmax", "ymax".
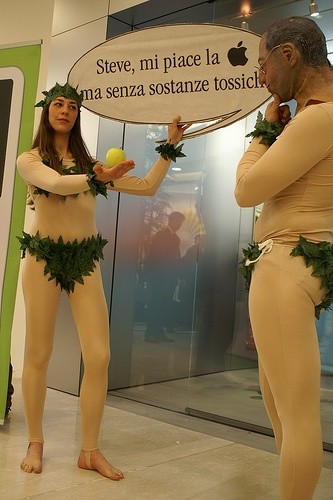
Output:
[{"xmin": 106, "ymin": 147, "xmax": 126, "ymax": 166}]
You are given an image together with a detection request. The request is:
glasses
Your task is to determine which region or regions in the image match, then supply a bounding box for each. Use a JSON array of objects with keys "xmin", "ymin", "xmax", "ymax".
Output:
[{"xmin": 256, "ymin": 43, "xmax": 281, "ymax": 73}]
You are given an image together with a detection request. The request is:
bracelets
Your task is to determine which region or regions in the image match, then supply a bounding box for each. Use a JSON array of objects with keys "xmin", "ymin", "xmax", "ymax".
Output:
[
  {"xmin": 154, "ymin": 139, "xmax": 187, "ymax": 163},
  {"xmin": 245, "ymin": 110, "xmax": 283, "ymax": 147},
  {"xmin": 85, "ymin": 160, "xmax": 115, "ymax": 201}
]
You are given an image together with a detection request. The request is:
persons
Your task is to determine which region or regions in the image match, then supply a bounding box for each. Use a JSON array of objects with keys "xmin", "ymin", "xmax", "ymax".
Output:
[
  {"xmin": 16, "ymin": 81, "xmax": 193, "ymax": 480},
  {"xmin": 234, "ymin": 16, "xmax": 333, "ymax": 500},
  {"xmin": 140, "ymin": 211, "xmax": 208, "ymax": 344}
]
